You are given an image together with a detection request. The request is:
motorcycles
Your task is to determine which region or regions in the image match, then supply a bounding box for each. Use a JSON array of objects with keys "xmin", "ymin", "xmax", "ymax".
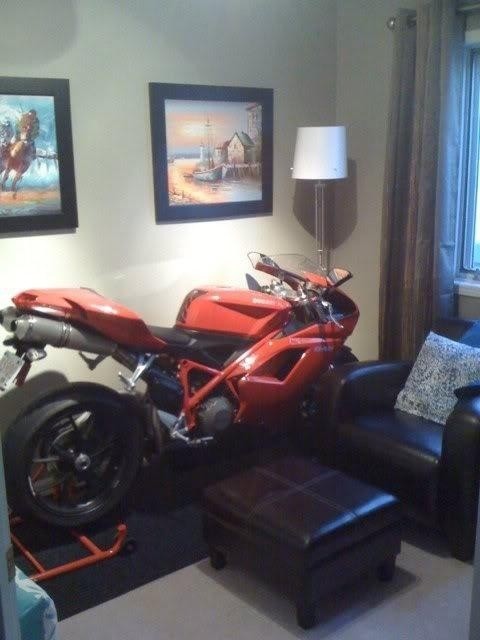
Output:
[{"xmin": 0, "ymin": 250, "xmax": 360, "ymax": 529}]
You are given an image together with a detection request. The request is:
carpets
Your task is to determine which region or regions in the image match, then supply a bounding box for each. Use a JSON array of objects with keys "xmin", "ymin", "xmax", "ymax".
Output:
[{"xmin": 10, "ymin": 464, "xmax": 210, "ymax": 623}]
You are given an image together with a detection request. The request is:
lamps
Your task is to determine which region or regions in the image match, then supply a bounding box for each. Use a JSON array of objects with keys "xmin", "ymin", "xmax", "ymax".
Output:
[{"xmin": 290, "ymin": 126, "xmax": 349, "ymax": 267}]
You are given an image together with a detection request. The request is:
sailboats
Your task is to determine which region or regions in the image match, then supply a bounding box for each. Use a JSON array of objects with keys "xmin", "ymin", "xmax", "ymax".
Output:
[{"xmin": 191, "ymin": 113, "xmax": 223, "ymax": 182}]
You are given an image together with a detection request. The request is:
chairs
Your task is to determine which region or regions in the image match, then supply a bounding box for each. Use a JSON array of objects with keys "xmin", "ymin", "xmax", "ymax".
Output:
[{"xmin": 313, "ymin": 322, "xmax": 480, "ymax": 561}]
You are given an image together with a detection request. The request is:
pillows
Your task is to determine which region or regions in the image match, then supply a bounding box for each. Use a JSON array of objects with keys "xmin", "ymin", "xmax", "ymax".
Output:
[{"xmin": 393, "ymin": 330, "xmax": 480, "ymax": 426}]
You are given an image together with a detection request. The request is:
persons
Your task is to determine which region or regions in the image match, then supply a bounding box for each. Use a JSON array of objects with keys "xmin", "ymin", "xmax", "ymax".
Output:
[{"xmin": 8, "ymin": 109, "xmax": 40, "ymax": 160}]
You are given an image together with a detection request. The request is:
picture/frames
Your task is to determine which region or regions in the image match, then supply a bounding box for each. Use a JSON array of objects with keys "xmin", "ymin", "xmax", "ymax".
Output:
[
  {"xmin": 0, "ymin": 76, "xmax": 79, "ymax": 233},
  {"xmin": 149, "ymin": 83, "xmax": 274, "ymax": 222}
]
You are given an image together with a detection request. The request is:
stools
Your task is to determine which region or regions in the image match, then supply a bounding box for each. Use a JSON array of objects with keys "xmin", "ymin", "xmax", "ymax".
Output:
[{"xmin": 199, "ymin": 455, "xmax": 402, "ymax": 630}]
